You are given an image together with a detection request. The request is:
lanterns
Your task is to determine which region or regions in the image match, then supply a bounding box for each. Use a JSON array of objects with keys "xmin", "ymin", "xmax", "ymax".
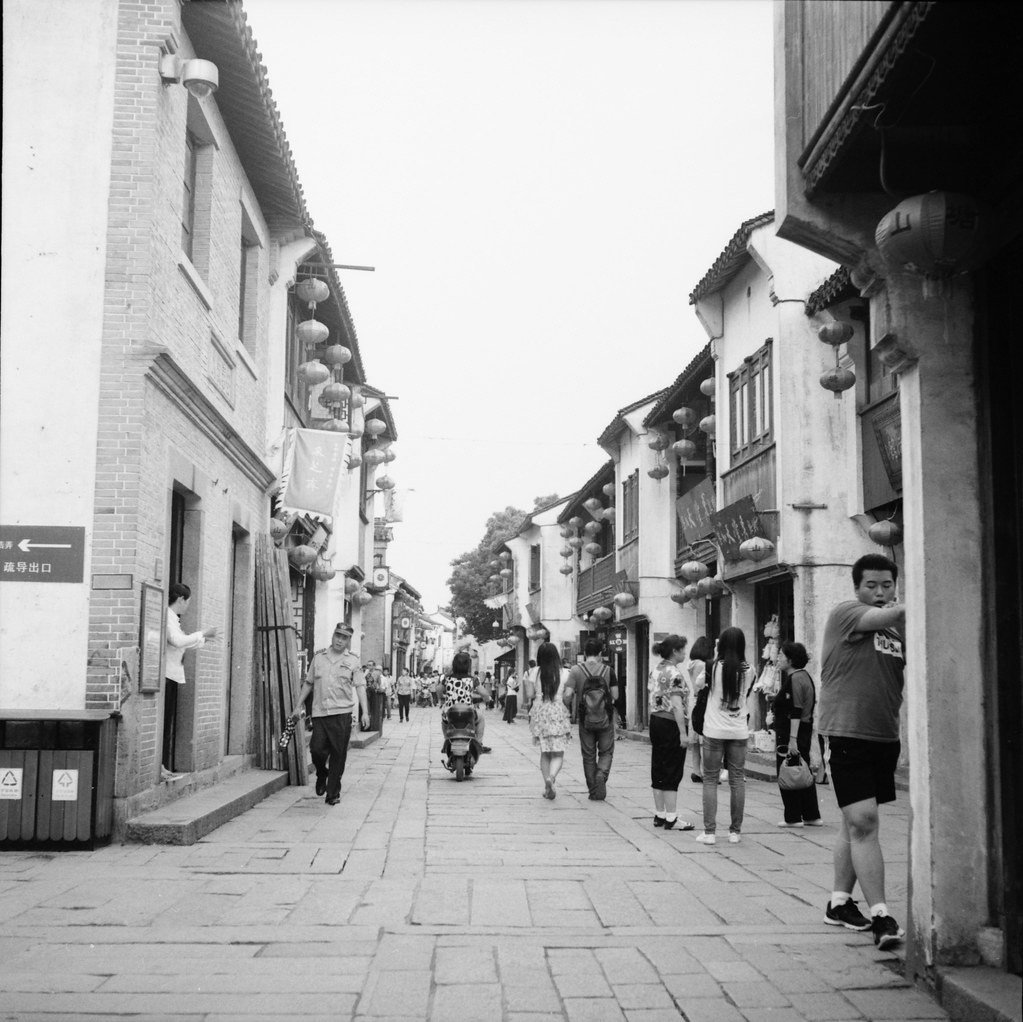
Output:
[
  {"xmin": 875, "ymin": 191, "xmax": 981, "ymax": 301},
  {"xmin": 868, "ymin": 520, "xmax": 902, "ymax": 548},
  {"xmin": 739, "ymin": 537, "xmax": 775, "ymax": 561},
  {"xmin": 488, "ymin": 377, "xmax": 730, "ymax": 648},
  {"xmin": 271, "ymin": 278, "xmax": 433, "ymax": 631},
  {"xmin": 817, "ymin": 319, "xmax": 854, "ymax": 401}
]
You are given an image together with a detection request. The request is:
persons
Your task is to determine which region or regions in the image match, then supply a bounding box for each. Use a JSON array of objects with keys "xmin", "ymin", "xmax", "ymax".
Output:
[
  {"xmin": 290, "ymin": 622, "xmax": 370, "ymax": 805},
  {"xmin": 381, "ymin": 667, "xmax": 396, "ymax": 721},
  {"xmin": 436, "ymin": 652, "xmax": 492, "ymax": 754},
  {"xmin": 502, "ymin": 670, "xmax": 521, "ymax": 724},
  {"xmin": 648, "ymin": 635, "xmax": 695, "ymax": 831},
  {"xmin": 562, "ymin": 658, "xmax": 569, "ymax": 668},
  {"xmin": 563, "ymin": 638, "xmax": 619, "ymax": 800},
  {"xmin": 526, "ymin": 642, "xmax": 573, "ymax": 800},
  {"xmin": 695, "ymin": 627, "xmax": 757, "ymax": 844},
  {"xmin": 410, "ymin": 670, "xmax": 446, "ymax": 709},
  {"xmin": 473, "ymin": 671, "xmax": 496, "ymax": 710},
  {"xmin": 393, "ymin": 668, "xmax": 414, "ymax": 723},
  {"xmin": 772, "ymin": 643, "xmax": 824, "ymax": 828},
  {"xmin": 364, "ymin": 660, "xmax": 381, "ymax": 691},
  {"xmin": 160, "ymin": 584, "xmax": 225, "ymax": 782},
  {"xmin": 687, "ymin": 636, "xmax": 722, "ymax": 785},
  {"xmin": 817, "ymin": 554, "xmax": 906, "ymax": 950},
  {"xmin": 523, "ymin": 660, "xmax": 536, "ymax": 725},
  {"xmin": 362, "ymin": 665, "xmax": 367, "ymax": 672}
]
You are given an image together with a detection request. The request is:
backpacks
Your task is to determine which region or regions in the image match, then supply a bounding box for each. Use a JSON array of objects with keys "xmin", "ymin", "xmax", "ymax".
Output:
[{"xmin": 577, "ymin": 662, "xmax": 613, "ymax": 731}]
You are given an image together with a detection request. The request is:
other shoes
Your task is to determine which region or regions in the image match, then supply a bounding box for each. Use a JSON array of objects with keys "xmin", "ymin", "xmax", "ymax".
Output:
[
  {"xmin": 778, "ymin": 821, "xmax": 803, "ymax": 827},
  {"xmin": 543, "ymin": 776, "xmax": 556, "ymax": 799},
  {"xmin": 162, "ymin": 772, "xmax": 182, "ymax": 782},
  {"xmin": 728, "ymin": 833, "xmax": 742, "ymax": 842},
  {"xmin": 441, "ymin": 747, "xmax": 448, "ymax": 753},
  {"xmin": 482, "ymin": 746, "xmax": 491, "ymax": 753},
  {"xmin": 589, "ymin": 772, "xmax": 606, "ymax": 800},
  {"xmin": 691, "ymin": 773, "xmax": 703, "ymax": 782},
  {"xmin": 696, "ymin": 830, "xmax": 715, "ymax": 845},
  {"xmin": 801, "ymin": 819, "xmax": 824, "ymax": 826}
]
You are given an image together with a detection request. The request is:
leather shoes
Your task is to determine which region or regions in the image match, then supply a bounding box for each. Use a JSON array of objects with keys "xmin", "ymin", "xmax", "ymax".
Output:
[
  {"xmin": 315, "ymin": 768, "xmax": 329, "ymax": 796},
  {"xmin": 326, "ymin": 794, "xmax": 340, "ymax": 804}
]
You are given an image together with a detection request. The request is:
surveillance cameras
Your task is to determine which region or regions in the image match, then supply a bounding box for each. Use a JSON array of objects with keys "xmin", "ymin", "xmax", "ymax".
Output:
[{"xmin": 182, "ymin": 59, "xmax": 219, "ymax": 101}]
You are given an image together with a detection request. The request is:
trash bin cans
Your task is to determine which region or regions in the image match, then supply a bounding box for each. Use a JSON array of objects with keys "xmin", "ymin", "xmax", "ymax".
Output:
[
  {"xmin": 0, "ymin": 707, "xmax": 123, "ymax": 852},
  {"xmin": 361, "ymin": 688, "xmax": 386, "ymax": 737}
]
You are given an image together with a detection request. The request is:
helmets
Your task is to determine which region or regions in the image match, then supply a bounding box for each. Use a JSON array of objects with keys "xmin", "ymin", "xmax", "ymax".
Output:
[{"xmin": 452, "ymin": 653, "xmax": 470, "ymax": 673}]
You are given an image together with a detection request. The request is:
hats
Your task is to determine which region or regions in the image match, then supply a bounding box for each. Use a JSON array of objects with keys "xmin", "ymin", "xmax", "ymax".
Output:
[{"xmin": 335, "ymin": 623, "xmax": 354, "ymax": 637}]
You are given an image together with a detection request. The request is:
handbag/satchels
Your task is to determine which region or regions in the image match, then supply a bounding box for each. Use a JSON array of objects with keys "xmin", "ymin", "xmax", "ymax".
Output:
[
  {"xmin": 692, "ymin": 659, "xmax": 714, "ymax": 736},
  {"xmin": 776, "ymin": 745, "xmax": 813, "ymax": 790}
]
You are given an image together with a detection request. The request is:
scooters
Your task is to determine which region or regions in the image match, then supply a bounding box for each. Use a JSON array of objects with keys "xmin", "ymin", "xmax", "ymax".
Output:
[{"xmin": 440, "ymin": 697, "xmax": 496, "ymax": 782}]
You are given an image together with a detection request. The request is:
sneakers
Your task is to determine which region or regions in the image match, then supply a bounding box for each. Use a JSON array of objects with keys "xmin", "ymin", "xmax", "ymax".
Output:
[
  {"xmin": 654, "ymin": 815, "xmax": 666, "ymax": 827},
  {"xmin": 664, "ymin": 816, "xmax": 695, "ymax": 830},
  {"xmin": 824, "ymin": 897, "xmax": 872, "ymax": 931},
  {"xmin": 871, "ymin": 911, "xmax": 905, "ymax": 950}
]
